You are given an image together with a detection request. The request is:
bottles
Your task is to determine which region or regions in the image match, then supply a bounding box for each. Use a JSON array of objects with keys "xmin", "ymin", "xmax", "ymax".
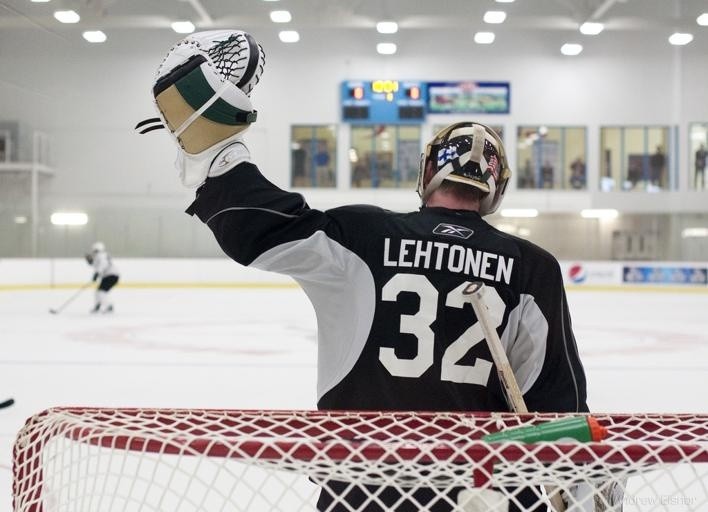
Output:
[{"xmin": 480, "ymin": 414, "xmax": 608, "ymax": 445}]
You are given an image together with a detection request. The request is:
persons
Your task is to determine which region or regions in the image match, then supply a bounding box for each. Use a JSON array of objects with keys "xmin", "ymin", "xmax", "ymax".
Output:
[
  {"xmin": 650, "ymin": 145, "xmax": 665, "ymax": 187},
  {"xmin": 292, "ymin": 142, "xmax": 307, "ymax": 187},
  {"xmin": 312, "ymin": 141, "xmax": 331, "ymax": 187},
  {"xmin": 151, "ymin": 28, "xmax": 633, "ymax": 512},
  {"xmin": 88, "ymin": 242, "xmax": 120, "ymax": 312},
  {"xmin": 568, "ymin": 157, "xmax": 587, "ymax": 188},
  {"xmin": 541, "ymin": 159, "xmax": 555, "ymax": 187},
  {"xmin": 693, "ymin": 143, "xmax": 708, "ymax": 189},
  {"xmin": 518, "ymin": 156, "xmax": 536, "ymax": 189}
]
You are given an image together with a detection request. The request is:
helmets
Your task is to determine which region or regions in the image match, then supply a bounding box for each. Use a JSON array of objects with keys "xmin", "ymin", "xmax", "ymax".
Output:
[
  {"xmin": 415, "ymin": 121, "xmax": 512, "ymax": 217},
  {"xmin": 92, "ymin": 241, "xmax": 104, "ymax": 253}
]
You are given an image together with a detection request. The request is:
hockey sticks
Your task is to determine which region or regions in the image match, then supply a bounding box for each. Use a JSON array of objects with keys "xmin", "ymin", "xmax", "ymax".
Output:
[{"xmin": 48, "ymin": 280, "xmax": 96, "ymax": 315}]
